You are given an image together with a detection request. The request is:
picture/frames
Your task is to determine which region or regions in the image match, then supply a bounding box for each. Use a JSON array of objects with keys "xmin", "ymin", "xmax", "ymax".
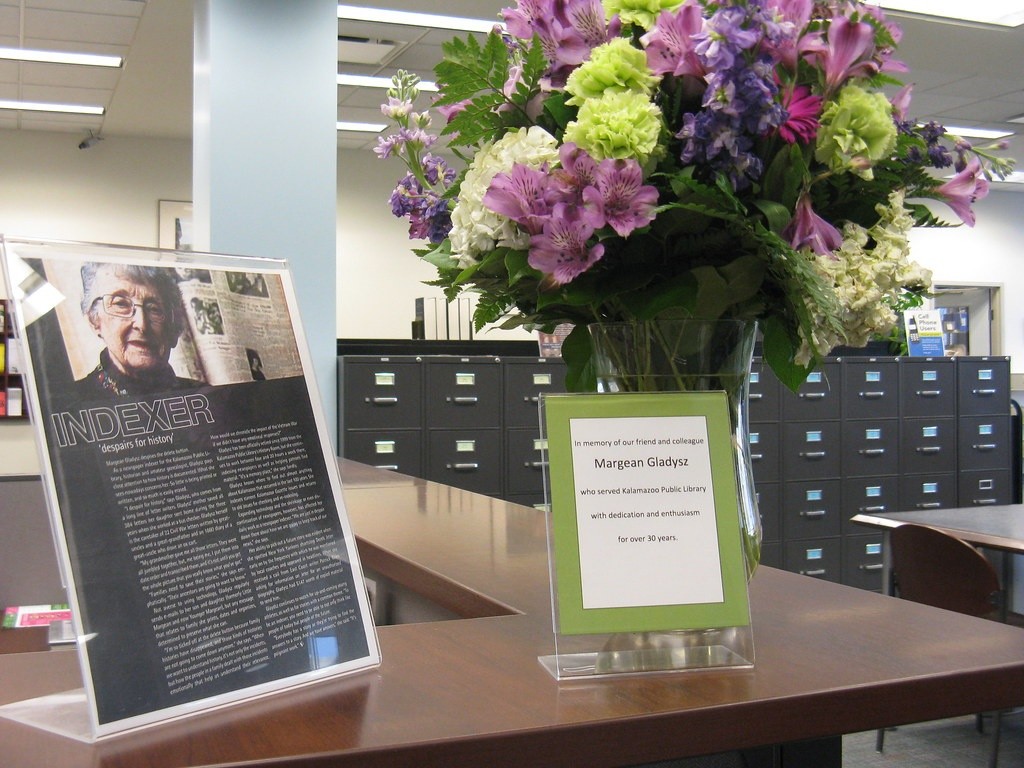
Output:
[{"xmin": 157, "ymin": 198, "xmax": 194, "ymax": 251}]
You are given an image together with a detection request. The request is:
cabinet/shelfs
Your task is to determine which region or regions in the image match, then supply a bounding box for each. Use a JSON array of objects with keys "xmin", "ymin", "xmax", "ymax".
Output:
[{"xmin": 336, "ymin": 353, "xmax": 1013, "ymax": 592}]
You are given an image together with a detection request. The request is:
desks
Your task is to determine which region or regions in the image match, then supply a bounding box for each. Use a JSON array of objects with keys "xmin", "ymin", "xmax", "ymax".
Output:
[{"xmin": 850, "ymin": 503, "xmax": 1024, "ymax": 597}]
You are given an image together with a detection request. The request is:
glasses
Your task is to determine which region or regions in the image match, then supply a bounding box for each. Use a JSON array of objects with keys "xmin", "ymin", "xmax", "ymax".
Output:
[{"xmin": 90, "ymin": 294, "xmax": 180, "ymax": 325}]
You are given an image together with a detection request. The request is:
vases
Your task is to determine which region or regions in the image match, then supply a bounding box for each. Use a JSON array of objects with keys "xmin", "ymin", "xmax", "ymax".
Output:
[{"xmin": 586, "ymin": 316, "xmax": 769, "ymax": 676}]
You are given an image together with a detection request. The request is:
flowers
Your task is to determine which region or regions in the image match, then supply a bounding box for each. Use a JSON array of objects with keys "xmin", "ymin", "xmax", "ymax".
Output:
[{"xmin": 370, "ymin": 0, "xmax": 1018, "ymax": 578}]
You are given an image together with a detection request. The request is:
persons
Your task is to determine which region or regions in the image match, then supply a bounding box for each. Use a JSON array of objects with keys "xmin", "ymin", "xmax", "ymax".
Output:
[
  {"xmin": 72, "ymin": 261, "xmax": 211, "ymax": 397},
  {"xmin": 233, "ymin": 272, "xmax": 268, "ymax": 298},
  {"xmin": 189, "ymin": 297, "xmax": 223, "ymax": 334},
  {"xmin": 247, "ymin": 348, "xmax": 265, "ymax": 380},
  {"xmin": 168, "ymin": 267, "xmax": 197, "ymax": 283}
]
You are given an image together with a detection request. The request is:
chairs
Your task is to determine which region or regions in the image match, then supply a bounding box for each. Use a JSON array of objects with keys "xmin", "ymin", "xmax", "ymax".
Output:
[{"xmin": 876, "ymin": 522, "xmax": 1010, "ymax": 768}]
